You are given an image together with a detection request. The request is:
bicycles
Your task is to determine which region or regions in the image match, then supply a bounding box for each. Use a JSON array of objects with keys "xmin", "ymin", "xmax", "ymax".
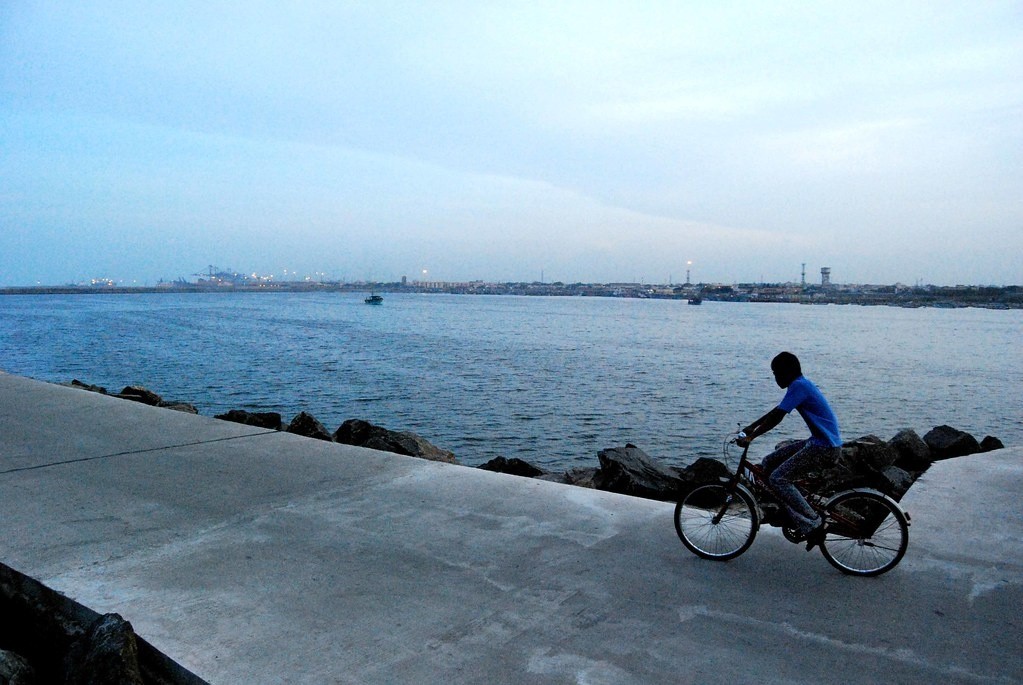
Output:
[{"xmin": 673, "ymin": 422, "xmax": 912, "ymax": 579}]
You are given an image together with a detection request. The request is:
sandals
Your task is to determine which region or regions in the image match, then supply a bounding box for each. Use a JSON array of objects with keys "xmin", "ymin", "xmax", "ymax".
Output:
[{"xmin": 805, "ymin": 521, "xmax": 832, "ymax": 552}]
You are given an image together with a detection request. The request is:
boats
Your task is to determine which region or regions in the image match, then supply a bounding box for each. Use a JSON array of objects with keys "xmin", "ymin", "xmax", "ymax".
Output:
[
  {"xmin": 687, "ymin": 297, "xmax": 702, "ymax": 305},
  {"xmin": 364, "ymin": 295, "xmax": 384, "ymax": 305}
]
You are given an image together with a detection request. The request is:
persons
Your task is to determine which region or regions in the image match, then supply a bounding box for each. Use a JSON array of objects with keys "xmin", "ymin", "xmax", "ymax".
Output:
[{"xmin": 735, "ymin": 350, "xmax": 842, "ymax": 552}]
